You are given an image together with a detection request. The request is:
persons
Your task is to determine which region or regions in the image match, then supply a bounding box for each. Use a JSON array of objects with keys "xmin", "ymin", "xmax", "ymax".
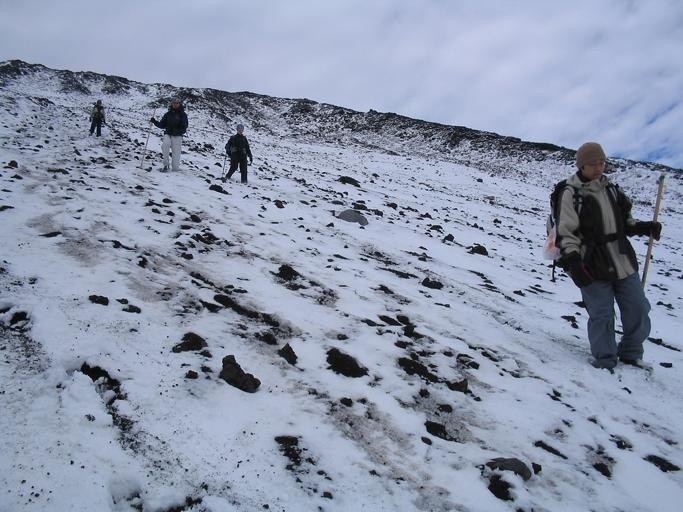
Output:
[
  {"xmin": 544, "ymin": 141, "xmax": 664, "ymax": 373},
  {"xmin": 87, "ymin": 105, "xmax": 101, "ymax": 137},
  {"xmin": 149, "ymin": 96, "xmax": 188, "ymax": 173},
  {"xmin": 223, "ymin": 123, "xmax": 252, "ymax": 184},
  {"xmin": 91, "ymin": 99, "xmax": 105, "ymax": 115}
]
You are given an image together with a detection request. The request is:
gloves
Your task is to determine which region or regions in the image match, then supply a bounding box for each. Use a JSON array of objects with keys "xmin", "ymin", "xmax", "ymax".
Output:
[
  {"xmin": 150, "ymin": 118, "xmax": 155, "ymax": 123},
  {"xmin": 250, "ymin": 157, "xmax": 252, "ymax": 163},
  {"xmin": 561, "ymin": 251, "xmax": 593, "ymax": 288},
  {"xmin": 636, "ymin": 220, "xmax": 662, "ymax": 241}
]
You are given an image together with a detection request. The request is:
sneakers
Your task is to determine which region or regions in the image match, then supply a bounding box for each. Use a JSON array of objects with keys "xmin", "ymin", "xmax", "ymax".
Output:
[
  {"xmin": 607, "ymin": 367, "xmax": 621, "ymax": 383},
  {"xmin": 619, "ymin": 357, "xmax": 652, "ymax": 370},
  {"xmin": 164, "ymin": 166, "xmax": 169, "ymax": 172}
]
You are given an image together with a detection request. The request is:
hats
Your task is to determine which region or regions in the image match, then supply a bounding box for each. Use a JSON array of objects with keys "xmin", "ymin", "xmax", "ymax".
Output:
[
  {"xmin": 577, "ymin": 143, "xmax": 606, "ymax": 167},
  {"xmin": 237, "ymin": 125, "xmax": 244, "ymax": 130}
]
